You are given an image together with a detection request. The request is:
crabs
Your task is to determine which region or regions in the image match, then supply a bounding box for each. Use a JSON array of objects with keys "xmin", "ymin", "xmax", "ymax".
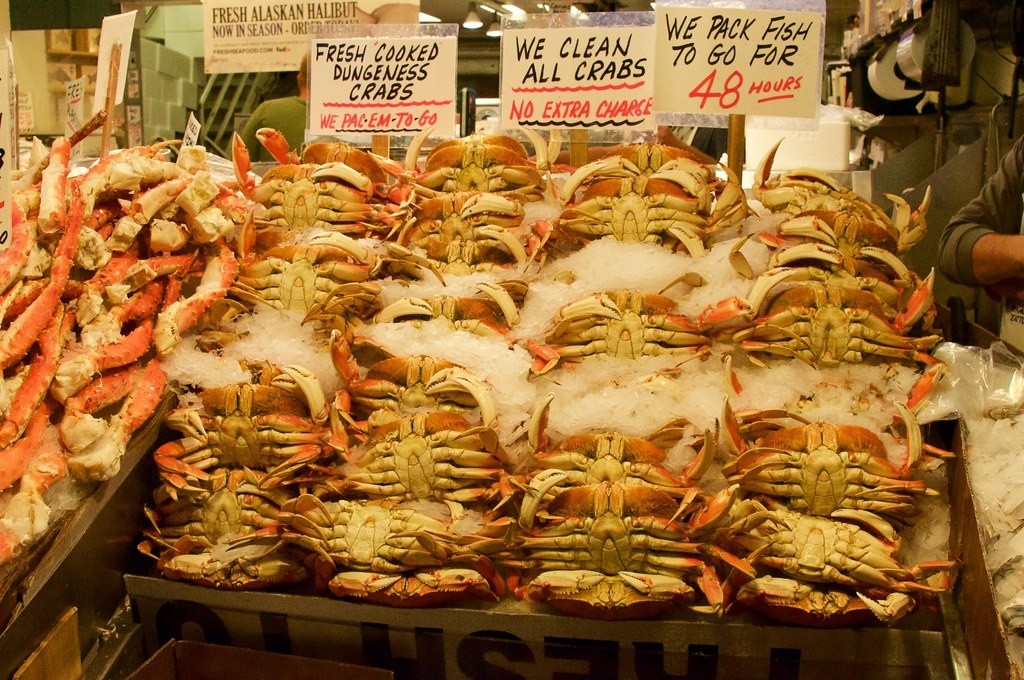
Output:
[{"xmin": 1, "ymin": 126, "xmax": 959, "ymax": 627}]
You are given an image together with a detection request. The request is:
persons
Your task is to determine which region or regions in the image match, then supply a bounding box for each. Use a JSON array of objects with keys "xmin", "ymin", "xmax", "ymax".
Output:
[
  {"xmin": 937, "ymin": 133, "xmax": 1024, "ymax": 357},
  {"xmin": 240, "ymin": 49, "xmax": 310, "ymax": 165}
]
